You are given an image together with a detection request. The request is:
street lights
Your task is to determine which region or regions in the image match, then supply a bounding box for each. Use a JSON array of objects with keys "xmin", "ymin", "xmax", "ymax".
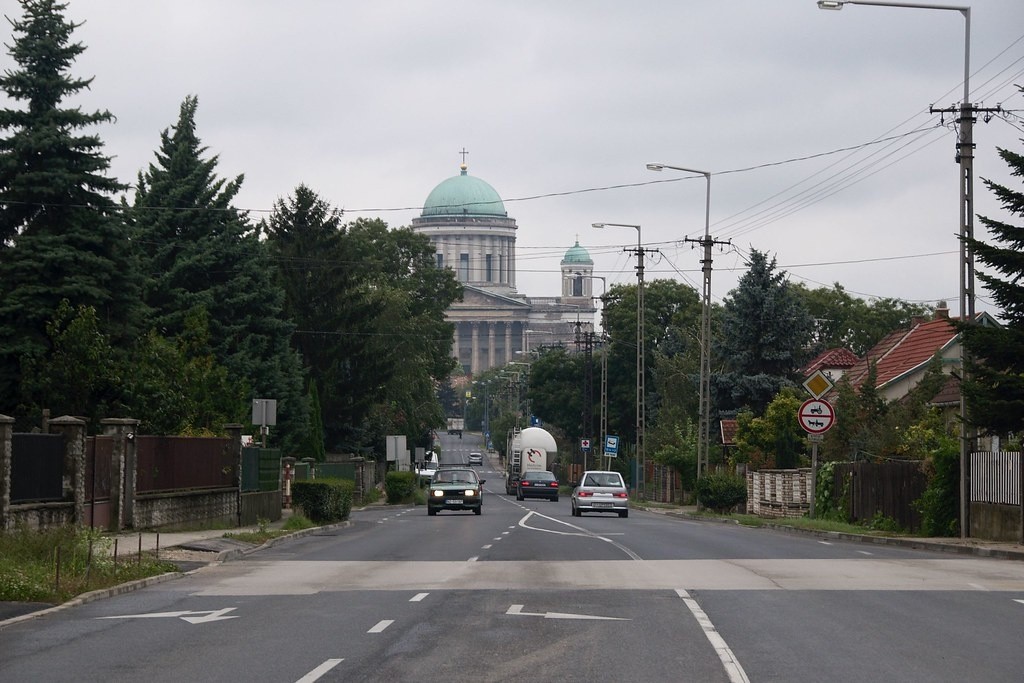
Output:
[
  {"xmin": 817, "ymin": 0, "xmax": 968, "ymax": 539},
  {"xmin": 567, "ymin": 274, "xmax": 608, "ymax": 471},
  {"xmin": 592, "ymin": 222, "xmax": 648, "ymax": 501},
  {"xmin": 647, "ymin": 164, "xmax": 711, "ymax": 511}
]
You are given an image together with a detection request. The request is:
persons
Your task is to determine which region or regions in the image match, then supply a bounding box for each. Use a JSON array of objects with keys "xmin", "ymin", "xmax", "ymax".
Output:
[{"xmin": 458, "ymin": 430, "xmax": 462, "ymax": 440}]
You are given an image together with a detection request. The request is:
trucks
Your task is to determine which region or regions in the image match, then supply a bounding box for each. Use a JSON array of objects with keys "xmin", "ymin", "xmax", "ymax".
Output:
[{"xmin": 447, "ymin": 418, "xmax": 463, "ymax": 435}]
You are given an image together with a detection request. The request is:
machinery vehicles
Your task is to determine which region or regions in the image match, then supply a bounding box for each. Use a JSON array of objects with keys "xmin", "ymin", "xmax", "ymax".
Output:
[{"xmin": 504, "ymin": 427, "xmax": 558, "ymax": 496}]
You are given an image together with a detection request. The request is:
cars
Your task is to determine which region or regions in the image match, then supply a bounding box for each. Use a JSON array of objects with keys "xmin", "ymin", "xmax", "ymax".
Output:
[
  {"xmin": 428, "ymin": 468, "xmax": 486, "ymax": 517},
  {"xmin": 516, "ymin": 471, "xmax": 558, "ymax": 502},
  {"xmin": 573, "ymin": 470, "xmax": 630, "ymax": 518},
  {"xmin": 469, "ymin": 453, "xmax": 482, "ymax": 467}
]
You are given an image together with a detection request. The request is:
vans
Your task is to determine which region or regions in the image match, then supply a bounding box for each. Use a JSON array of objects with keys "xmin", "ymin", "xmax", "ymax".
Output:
[{"xmin": 415, "ymin": 451, "xmax": 438, "ymax": 483}]
